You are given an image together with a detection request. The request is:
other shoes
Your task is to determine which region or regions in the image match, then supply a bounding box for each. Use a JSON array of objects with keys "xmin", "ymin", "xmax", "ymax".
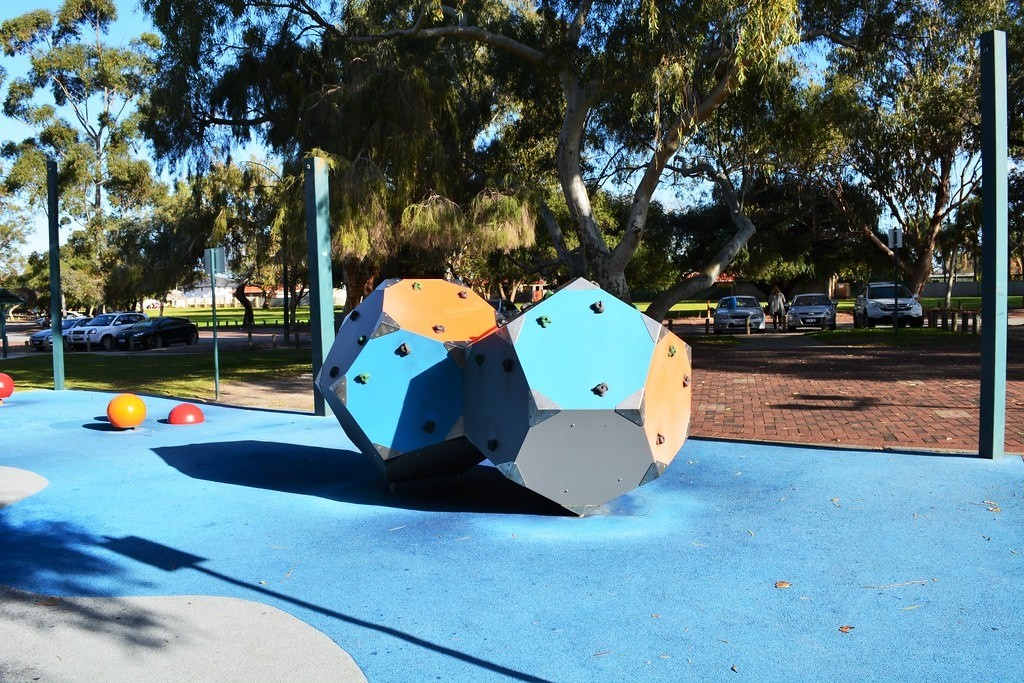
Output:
[{"xmin": 773, "ymin": 326, "xmax": 783, "ymax": 331}]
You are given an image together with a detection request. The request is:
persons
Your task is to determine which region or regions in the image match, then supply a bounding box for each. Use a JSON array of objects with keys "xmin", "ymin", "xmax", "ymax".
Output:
[
  {"xmin": 160, "ymin": 302, "xmax": 163, "ymax": 317},
  {"xmin": 768, "ymin": 286, "xmax": 786, "ymax": 332}
]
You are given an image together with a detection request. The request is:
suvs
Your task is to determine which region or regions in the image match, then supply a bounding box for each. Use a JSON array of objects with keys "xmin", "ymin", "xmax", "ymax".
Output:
[
  {"xmin": 853, "ymin": 282, "xmax": 924, "ymax": 330},
  {"xmin": 67, "ymin": 310, "xmax": 153, "ymax": 350}
]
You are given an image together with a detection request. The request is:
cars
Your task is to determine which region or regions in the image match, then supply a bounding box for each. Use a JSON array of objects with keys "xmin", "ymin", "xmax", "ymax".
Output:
[
  {"xmin": 115, "ymin": 316, "xmax": 199, "ymax": 350},
  {"xmin": 783, "ymin": 293, "xmax": 838, "ymax": 332},
  {"xmin": 710, "ymin": 296, "xmax": 769, "ymax": 333},
  {"xmin": 35, "ymin": 311, "xmax": 88, "ymax": 328},
  {"xmin": 29, "ymin": 317, "xmax": 107, "ymax": 351},
  {"xmin": 485, "ymin": 298, "xmax": 520, "ymax": 317}
]
